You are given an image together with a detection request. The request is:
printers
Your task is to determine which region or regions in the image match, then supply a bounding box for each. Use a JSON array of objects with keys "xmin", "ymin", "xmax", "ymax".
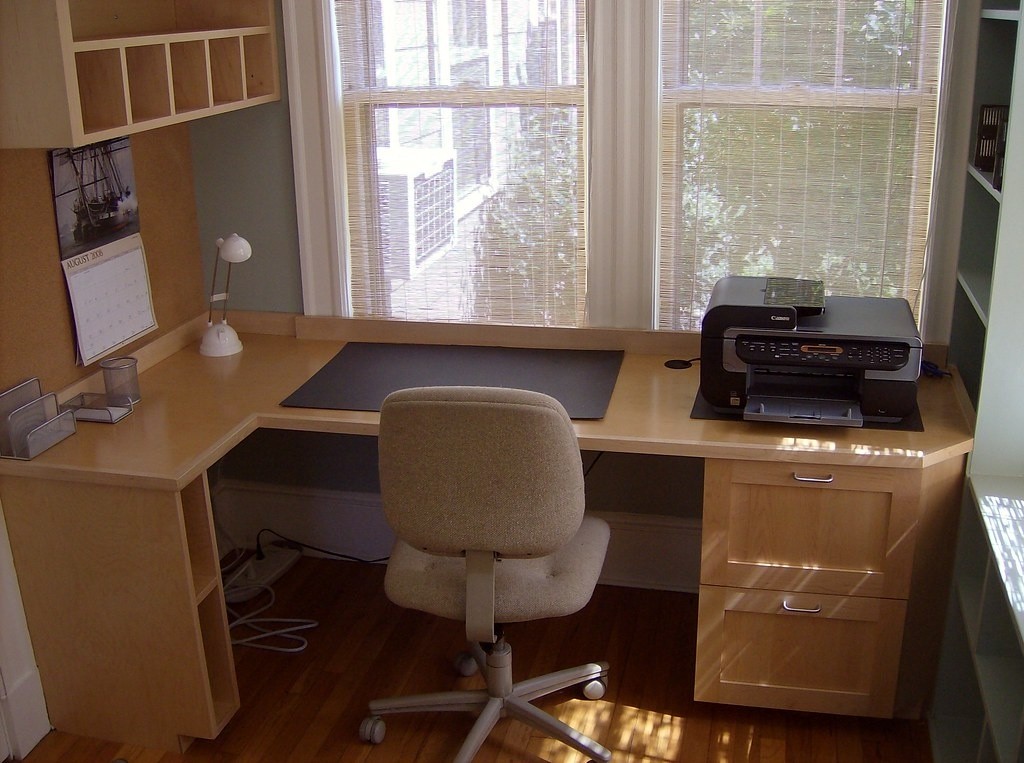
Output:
[{"xmin": 700, "ymin": 275, "xmax": 923, "ymax": 429}]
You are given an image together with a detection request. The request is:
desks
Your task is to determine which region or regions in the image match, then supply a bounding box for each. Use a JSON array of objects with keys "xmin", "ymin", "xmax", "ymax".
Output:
[{"xmin": 0, "ymin": 334, "xmax": 974, "ymax": 760}]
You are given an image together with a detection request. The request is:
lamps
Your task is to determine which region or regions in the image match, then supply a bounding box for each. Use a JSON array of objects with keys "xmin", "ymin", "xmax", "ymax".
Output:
[{"xmin": 199, "ymin": 232, "xmax": 252, "ymax": 357}]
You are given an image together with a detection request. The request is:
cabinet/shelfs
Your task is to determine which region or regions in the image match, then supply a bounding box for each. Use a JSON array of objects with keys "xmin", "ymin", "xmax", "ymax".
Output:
[
  {"xmin": 931, "ymin": 474, "xmax": 1024, "ymax": 763},
  {"xmin": 947, "ymin": 0, "xmax": 1023, "ymax": 472},
  {"xmin": 0, "ymin": 0, "xmax": 281, "ymax": 150},
  {"xmin": 694, "ymin": 457, "xmax": 968, "ymax": 720}
]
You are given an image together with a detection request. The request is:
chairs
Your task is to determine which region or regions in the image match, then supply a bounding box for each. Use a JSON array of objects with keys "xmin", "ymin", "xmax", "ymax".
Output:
[{"xmin": 360, "ymin": 384, "xmax": 615, "ymax": 763}]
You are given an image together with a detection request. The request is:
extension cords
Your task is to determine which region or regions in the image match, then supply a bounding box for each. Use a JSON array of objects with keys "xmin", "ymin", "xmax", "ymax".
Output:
[{"xmin": 226, "ymin": 539, "xmax": 303, "ymax": 601}]
